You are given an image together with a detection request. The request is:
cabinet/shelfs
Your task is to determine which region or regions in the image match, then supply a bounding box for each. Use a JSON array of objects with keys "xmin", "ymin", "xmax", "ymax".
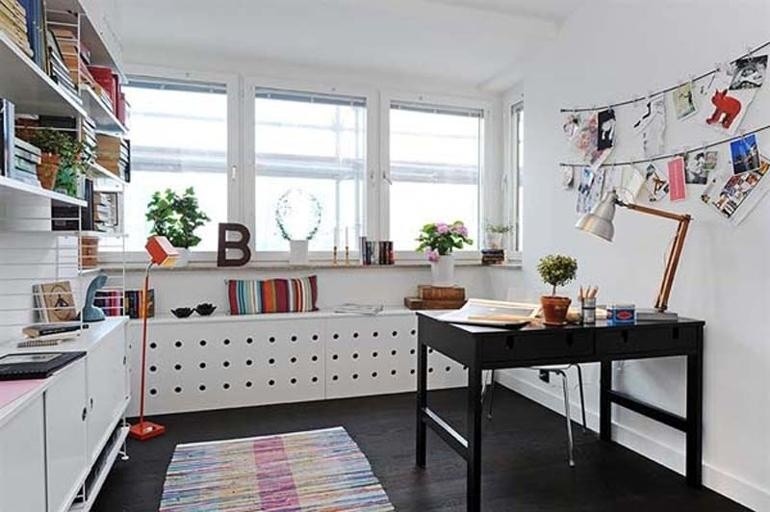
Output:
[
  {"xmin": 0, "ymin": 321, "xmax": 128, "ymax": 512},
  {"xmin": 0, "ymin": 0, "xmax": 128, "ymax": 238}
]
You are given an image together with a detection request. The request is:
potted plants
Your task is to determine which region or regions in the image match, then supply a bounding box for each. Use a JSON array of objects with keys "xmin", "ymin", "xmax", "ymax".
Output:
[
  {"xmin": 276, "ymin": 186, "xmax": 324, "ymax": 266},
  {"xmin": 479, "ymin": 219, "xmax": 515, "ymax": 264},
  {"xmin": 537, "ymin": 254, "xmax": 578, "ymax": 323},
  {"xmin": 146, "ymin": 186, "xmax": 211, "ymax": 266}
]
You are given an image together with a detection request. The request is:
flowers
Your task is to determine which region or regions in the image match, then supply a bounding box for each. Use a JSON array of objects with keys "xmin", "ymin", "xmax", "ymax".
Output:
[{"xmin": 414, "ymin": 220, "xmax": 471, "ymax": 264}]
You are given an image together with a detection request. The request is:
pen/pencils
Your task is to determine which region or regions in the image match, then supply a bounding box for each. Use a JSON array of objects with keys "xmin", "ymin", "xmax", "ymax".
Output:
[{"xmin": 577, "ymin": 285, "xmax": 598, "ymax": 298}]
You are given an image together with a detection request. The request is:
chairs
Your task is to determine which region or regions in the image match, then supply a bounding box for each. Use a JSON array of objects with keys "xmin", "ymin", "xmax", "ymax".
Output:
[{"xmin": 481, "ymin": 287, "xmax": 585, "ymax": 467}]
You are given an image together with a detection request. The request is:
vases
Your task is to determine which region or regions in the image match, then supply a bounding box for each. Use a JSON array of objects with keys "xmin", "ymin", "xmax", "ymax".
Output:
[{"xmin": 431, "ymin": 253, "xmax": 455, "ymax": 289}]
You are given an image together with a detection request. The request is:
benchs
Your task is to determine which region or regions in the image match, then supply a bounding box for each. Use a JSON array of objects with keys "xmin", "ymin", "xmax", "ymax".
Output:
[
  {"xmin": 325, "ymin": 308, "xmax": 493, "ymax": 402},
  {"xmin": 129, "ymin": 306, "xmax": 326, "ymax": 415}
]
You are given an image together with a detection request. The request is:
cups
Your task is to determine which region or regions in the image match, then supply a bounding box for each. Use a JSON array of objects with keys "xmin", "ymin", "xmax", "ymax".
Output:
[{"xmin": 577, "ymin": 297, "xmax": 597, "ymax": 324}]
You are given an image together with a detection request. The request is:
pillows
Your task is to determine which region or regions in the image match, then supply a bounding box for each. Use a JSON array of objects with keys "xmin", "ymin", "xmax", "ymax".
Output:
[{"xmin": 224, "ymin": 275, "xmax": 319, "ymax": 314}]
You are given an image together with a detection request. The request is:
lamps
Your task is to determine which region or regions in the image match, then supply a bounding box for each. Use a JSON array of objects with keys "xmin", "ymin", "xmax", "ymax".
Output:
[
  {"xmin": 574, "ymin": 188, "xmax": 691, "ymax": 321},
  {"xmin": 129, "ymin": 236, "xmax": 180, "ymax": 441}
]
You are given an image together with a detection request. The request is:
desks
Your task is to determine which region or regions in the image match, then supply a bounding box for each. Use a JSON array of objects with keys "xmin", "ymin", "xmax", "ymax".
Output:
[{"xmin": 415, "ymin": 305, "xmax": 705, "ymax": 512}]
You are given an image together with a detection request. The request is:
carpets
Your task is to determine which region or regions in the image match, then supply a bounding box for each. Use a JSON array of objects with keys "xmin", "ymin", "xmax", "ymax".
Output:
[{"xmin": 159, "ymin": 426, "xmax": 396, "ymax": 512}]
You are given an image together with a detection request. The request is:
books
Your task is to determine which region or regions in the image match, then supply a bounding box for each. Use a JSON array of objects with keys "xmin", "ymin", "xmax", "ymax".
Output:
[
  {"xmin": 358, "ymin": 237, "xmax": 395, "ymax": 266},
  {"xmin": 0, "ymin": 278, "xmax": 91, "ymax": 378},
  {"xmin": 336, "ymin": 302, "xmax": 385, "ymax": 316},
  {"xmin": 1, "ymin": 1, "xmax": 132, "ymax": 230},
  {"xmin": 92, "ymin": 286, "xmax": 155, "ymax": 318}
]
